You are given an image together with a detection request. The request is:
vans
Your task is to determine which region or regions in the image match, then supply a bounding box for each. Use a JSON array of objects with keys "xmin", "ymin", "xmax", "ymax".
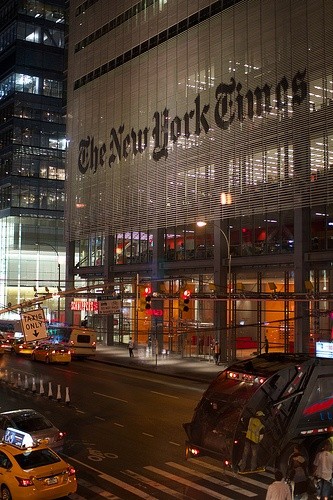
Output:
[{"xmin": 49, "ymin": 327, "xmax": 99, "ymax": 360}]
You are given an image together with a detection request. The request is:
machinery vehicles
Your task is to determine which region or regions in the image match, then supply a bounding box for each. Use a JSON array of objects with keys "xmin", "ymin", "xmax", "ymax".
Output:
[{"xmin": 186, "ymin": 351, "xmax": 333, "ymax": 475}]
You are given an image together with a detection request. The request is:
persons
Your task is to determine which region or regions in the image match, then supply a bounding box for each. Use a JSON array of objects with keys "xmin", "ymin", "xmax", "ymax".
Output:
[
  {"xmin": 265, "ymin": 471, "xmax": 292, "ymax": 500},
  {"xmin": 213, "ymin": 339, "xmax": 220, "ymax": 365},
  {"xmin": 287, "ymin": 431, "xmax": 333, "ymax": 500},
  {"xmin": 240, "ymin": 410, "xmax": 266, "ymax": 471},
  {"xmin": 126, "ymin": 336, "xmax": 134, "ymax": 358},
  {"xmin": 262, "ymin": 335, "xmax": 269, "ymax": 353}
]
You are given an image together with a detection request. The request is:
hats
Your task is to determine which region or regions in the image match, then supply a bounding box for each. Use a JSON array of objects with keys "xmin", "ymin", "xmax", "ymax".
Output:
[{"xmin": 255, "ymin": 411, "xmax": 265, "ymax": 416}]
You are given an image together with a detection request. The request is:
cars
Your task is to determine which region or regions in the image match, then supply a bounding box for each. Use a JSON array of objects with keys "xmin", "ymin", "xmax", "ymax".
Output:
[
  {"xmin": 1, "ymin": 410, "xmax": 66, "ymax": 454},
  {"xmin": 11, "ymin": 339, "xmax": 37, "ymax": 354},
  {"xmin": 1, "ymin": 337, "xmax": 13, "ymax": 352},
  {"xmin": 0, "ymin": 428, "xmax": 77, "ymax": 500},
  {"xmin": 33, "ymin": 344, "xmax": 71, "ymax": 364}
]
